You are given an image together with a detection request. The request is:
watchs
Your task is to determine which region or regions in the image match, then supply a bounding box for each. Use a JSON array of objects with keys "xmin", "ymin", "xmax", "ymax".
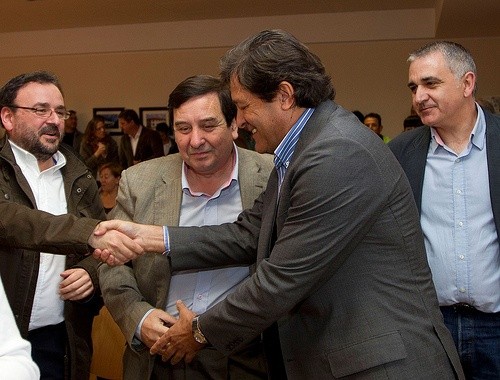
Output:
[{"xmin": 191, "ymin": 314, "xmax": 210, "ymax": 345}]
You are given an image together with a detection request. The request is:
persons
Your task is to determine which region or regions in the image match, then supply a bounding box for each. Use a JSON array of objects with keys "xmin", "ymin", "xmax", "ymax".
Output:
[
  {"xmin": 494, "ymin": 100, "xmax": 500, "ymax": 116},
  {"xmin": 386, "ymin": 39, "xmax": 500, "ymax": 380},
  {"xmin": 0, "ymin": 273, "xmax": 42, "ymax": 380},
  {"xmin": 98, "ymin": 163, "xmax": 122, "ymax": 215},
  {"xmin": 0, "ymin": 70, "xmax": 145, "ymax": 380},
  {"xmin": 155, "ymin": 122, "xmax": 179, "ymax": 157},
  {"xmin": 475, "ymin": 99, "xmax": 496, "ymax": 115},
  {"xmin": 363, "ymin": 112, "xmax": 392, "ymax": 144},
  {"xmin": 117, "ymin": 108, "xmax": 165, "ymax": 170},
  {"xmin": 410, "ymin": 103, "xmax": 418, "ymax": 114},
  {"xmin": 80, "ymin": 117, "xmax": 118, "ymax": 179},
  {"xmin": 91, "ymin": 27, "xmax": 468, "ymax": 380},
  {"xmin": 97, "ymin": 74, "xmax": 288, "ymax": 380},
  {"xmin": 402, "ymin": 115, "xmax": 424, "ymax": 132},
  {"xmin": 64, "ymin": 108, "xmax": 84, "ymax": 156},
  {"xmin": 350, "ymin": 109, "xmax": 364, "ymax": 123}
]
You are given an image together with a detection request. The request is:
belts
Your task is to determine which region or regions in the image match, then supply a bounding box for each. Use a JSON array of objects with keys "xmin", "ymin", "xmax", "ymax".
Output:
[{"xmin": 440, "ymin": 302, "xmax": 500, "ymax": 320}]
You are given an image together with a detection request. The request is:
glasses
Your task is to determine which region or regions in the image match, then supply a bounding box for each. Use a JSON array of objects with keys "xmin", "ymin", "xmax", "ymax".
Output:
[{"xmin": 8, "ymin": 105, "xmax": 71, "ymax": 120}]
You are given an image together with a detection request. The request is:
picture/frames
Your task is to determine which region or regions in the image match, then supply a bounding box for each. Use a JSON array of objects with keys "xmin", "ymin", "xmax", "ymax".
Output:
[
  {"xmin": 139, "ymin": 107, "xmax": 172, "ymax": 134},
  {"xmin": 93, "ymin": 108, "xmax": 124, "ymax": 136}
]
